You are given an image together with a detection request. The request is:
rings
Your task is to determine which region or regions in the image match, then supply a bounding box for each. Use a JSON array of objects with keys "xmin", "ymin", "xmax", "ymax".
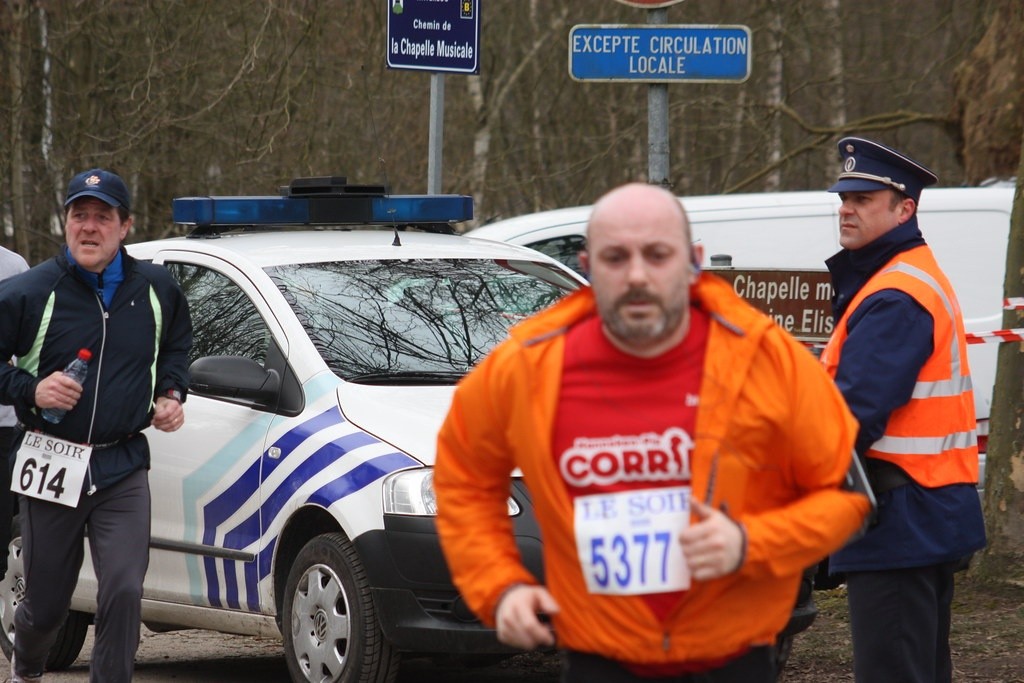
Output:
[{"xmin": 175, "ymin": 418, "xmax": 178, "ymax": 423}]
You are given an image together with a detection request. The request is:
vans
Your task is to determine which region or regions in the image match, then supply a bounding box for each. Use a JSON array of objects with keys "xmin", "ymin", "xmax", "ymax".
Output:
[{"xmin": 454, "ymin": 178, "xmax": 1017, "ymax": 512}]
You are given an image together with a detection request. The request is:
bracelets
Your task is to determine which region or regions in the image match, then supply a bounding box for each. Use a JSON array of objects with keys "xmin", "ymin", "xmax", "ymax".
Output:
[{"xmin": 163, "ymin": 389, "xmax": 181, "ymax": 404}]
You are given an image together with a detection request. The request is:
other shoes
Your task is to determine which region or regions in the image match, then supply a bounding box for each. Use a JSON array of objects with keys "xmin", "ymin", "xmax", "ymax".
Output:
[{"xmin": 13, "ymin": 648, "xmax": 45, "ymax": 682}]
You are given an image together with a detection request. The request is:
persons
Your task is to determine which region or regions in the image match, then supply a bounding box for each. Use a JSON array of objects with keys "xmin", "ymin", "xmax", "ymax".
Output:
[
  {"xmin": 0, "ymin": 168, "xmax": 192, "ymax": 683},
  {"xmin": 819, "ymin": 137, "xmax": 989, "ymax": 683},
  {"xmin": 432, "ymin": 182, "xmax": 871, "ymax": 682}
]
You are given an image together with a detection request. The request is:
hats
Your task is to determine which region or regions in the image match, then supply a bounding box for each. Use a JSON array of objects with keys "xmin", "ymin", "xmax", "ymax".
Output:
[
  {"xmin": 63, "ymin": 168, "xmax": 132, "ymax": 210},
  {"xmin": 827, "ymin": 135, "xmax": 938, "ymax": 203}
]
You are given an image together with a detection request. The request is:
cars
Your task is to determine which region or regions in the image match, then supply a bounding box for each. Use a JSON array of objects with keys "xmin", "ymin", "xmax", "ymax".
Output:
[{"xmin": 1, "ymin": 173, "xmax": 824, "ymax": 683}]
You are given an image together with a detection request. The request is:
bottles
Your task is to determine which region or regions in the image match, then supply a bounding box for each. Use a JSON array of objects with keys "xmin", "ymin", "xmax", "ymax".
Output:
[{"xmin": 43, "ymin": 349, "xmax": 92, "ymax": 424}]
